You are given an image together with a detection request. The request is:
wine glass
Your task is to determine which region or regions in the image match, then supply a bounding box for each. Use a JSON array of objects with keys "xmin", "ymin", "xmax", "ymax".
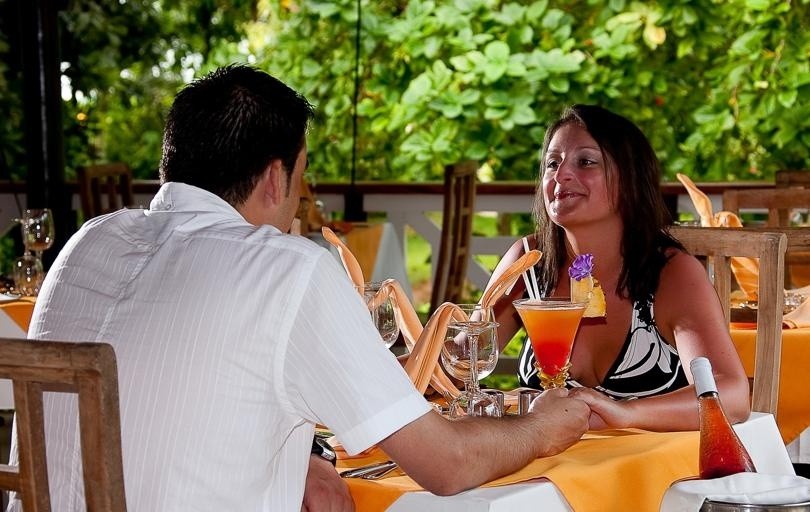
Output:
[
  {"xmin": 445, "ymin": 321, "xmax": 504, "ymax": 420},
  {"xmin": 439, "ymin": 299, "xmax": 501, "ymax": 391},
  {"xmin": 19, "ymin": 207, "xmax": 56, "ymax": 263}
]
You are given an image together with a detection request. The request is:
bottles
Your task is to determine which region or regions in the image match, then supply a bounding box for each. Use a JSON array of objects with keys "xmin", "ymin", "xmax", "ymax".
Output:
[{"xmin": 685, "ymin": 357, "xmax": 760, "ymax": 485}]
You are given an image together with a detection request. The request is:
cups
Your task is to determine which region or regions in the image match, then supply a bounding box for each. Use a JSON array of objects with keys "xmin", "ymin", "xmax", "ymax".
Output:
[
  {"xmin": 10, "ymin": 216, "xmax": 43, "ymax": 295},
  {"xmin": 354, "ymin": 281, "xmax": 400, "ymax": 347},
  {"xmin": 513, "ymin": 296, "xmax": 586, "ymax": 387}
]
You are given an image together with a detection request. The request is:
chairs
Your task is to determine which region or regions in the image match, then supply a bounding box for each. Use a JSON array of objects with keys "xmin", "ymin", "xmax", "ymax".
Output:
[
  {"xmin": 76, "ymin": 163, "xmax": 136, "ymax": 220},
  {"xmin": 1, "ymin": 334, "xmax": 128, "ymax": 512},
  {"xmin": 428, "ymin": 157, "xmax": 810, "ymax": 422}
]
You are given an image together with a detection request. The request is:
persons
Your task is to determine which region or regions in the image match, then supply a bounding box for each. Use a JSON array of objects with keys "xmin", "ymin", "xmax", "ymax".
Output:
[
  {"xmin": 429, "ymin": 102, "xmax": 752, "ymax": 437},
  {"xmin": 2, "ymin": 63, "xmax": 593, "ymax": 512}
]
